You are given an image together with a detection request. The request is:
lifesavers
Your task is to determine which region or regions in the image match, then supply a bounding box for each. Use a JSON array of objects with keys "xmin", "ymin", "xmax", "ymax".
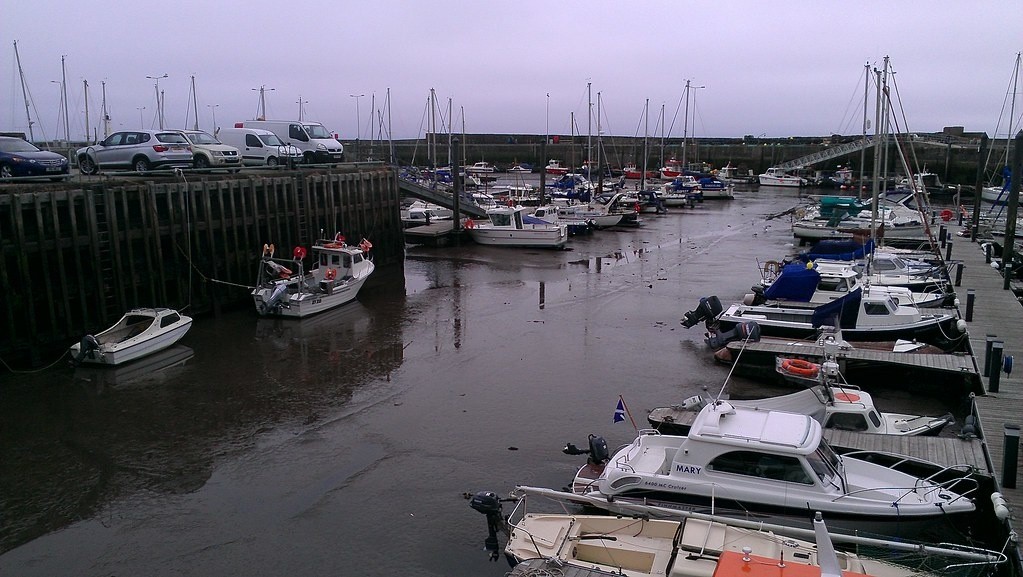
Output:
[
  {"xmin": 797, "ymin": 209, "xmax": 806, "ymax": 217},
  {"xmin": 765, "ymin": 260, "xmax": 778, "ymax": 273},
  {"xmin": 782, "ymin": 358, "xmax": 819, "ymax": 377},
  {"xmin": 693, "ymin": 186, "xmax": 699, "ymax": 191},
  {"xmin": 466, "ymin": 219, "xmax": 474, "ymax": 229},
  {"xmin": 324, "ymin": 243, "xmax": 343, "ymax": 249}
]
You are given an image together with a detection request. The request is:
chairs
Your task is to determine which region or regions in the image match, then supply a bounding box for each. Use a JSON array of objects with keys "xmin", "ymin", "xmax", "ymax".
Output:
[{"xmin": 127, "ymin": 137, "xmax": 134, "ymax": 144}]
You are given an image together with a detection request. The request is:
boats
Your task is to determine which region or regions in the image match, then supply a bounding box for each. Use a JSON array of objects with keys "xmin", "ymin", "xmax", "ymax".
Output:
[
  {"xmin": 641, "ymin": 187, "xmax": 688, "ymax": 213},
  {"xmin": 398, "ymin": 198, "xmax": 464, "ymax": 220},
  {"xmin": 758, "ymin": 168, "xmax": 802, "ymax": 187},
  {"xmin": 709, "ymin": 243, "xmax": 959, "ymax": 347},
  {"xmin": 895, "ymin": 172, "xmax": 955, "ymax": 205},
  {"xmin": 982, "ymin": 186, "xmax": 1023, "ymax": 204},
  {"xmin": 665, "ymin": 176, "xmax": 735, "ymax": 203},
  {"xmin": 399, "ymin": 158, "xmax": 757, "ymax": 205},
  {"xmin": 69, "ymin": 306, "xmax": 193, "ymax": 367},
  {"xmin": 251, "ymin": 237, "xmax": 376, "ymax": 321},
  {"xmin": 695, "ymin": 385, "xmax": 949, "ymax": 437},
  {"xmin": 466, "ymin": 204, "xmax": 571, "ymax": 249},
  {"xmin": 555, "ymin": 193, "xmax": 640, "ymax": 227},
  {"xmin": 492, "ymin": 405, "xmax": 980, "ymax": 577},
  {"xmin": 793, "ymin": 190, "xmax": 931, "ymax": 243},
  {"xmin": 520, "ymin": 207, "xmax": 595, "ymax": 233}
]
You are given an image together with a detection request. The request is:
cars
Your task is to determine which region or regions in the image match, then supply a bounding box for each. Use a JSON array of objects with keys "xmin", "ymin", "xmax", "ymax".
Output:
[
  {"xmin": 175, "ymin": 129, "xmax": 245, "ymax": 174},
  {"xmin": 0, "ymin": 134, "xmax": 69, "ymax": 177},
  {"xmin": 218, "ymin": 128, "xmax": 304, "ymax": 169},
  {"xmin": 74, "ymin": 129, "xmax": 195, "ymax": 175},
  {"xmin": 243, "ymin": 120, "xmax": 345, "ymax": 165}
]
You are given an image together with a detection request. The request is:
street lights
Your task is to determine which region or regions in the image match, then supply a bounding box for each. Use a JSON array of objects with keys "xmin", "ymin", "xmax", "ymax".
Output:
[
  {"xmin": 349, "ymin": 94, "xmax": 367, "ymax": 141},
  {"xmin": 146, "ymin": 75, "xmax": 170, "ymax": 129},
  {"xmin": 252, "ymin": 88, "xmax": 275, "ymax": 121}
]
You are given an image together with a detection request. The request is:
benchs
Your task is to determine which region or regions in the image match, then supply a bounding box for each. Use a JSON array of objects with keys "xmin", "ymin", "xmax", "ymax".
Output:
[{"xmin": 567, "ymin": 532, "xmax": 673, "ymax": 577}]
